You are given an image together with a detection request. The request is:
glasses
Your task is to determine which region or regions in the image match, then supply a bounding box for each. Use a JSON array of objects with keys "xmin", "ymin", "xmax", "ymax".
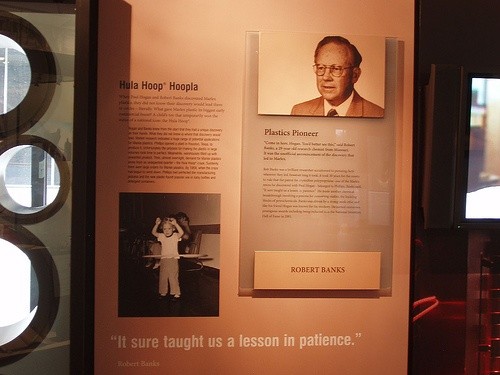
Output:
[{"xmin": 312, "ymin": 63, "xmax": 354, "ymax": 78}]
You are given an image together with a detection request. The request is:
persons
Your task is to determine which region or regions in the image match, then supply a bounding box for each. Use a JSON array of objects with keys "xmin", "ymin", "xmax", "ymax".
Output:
[
  {"xmin": 291, "ymin": 36, "xmax": 385, "ymax": 118},
  {"xmin": 153, "ymin": 217, "xmax": 184, "ymax": 303}
]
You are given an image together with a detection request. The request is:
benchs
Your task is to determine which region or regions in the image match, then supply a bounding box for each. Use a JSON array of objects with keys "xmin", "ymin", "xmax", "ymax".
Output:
[{"xmin": 140, "ymin": 227, "xmax": 205, "ymax": 272}]
